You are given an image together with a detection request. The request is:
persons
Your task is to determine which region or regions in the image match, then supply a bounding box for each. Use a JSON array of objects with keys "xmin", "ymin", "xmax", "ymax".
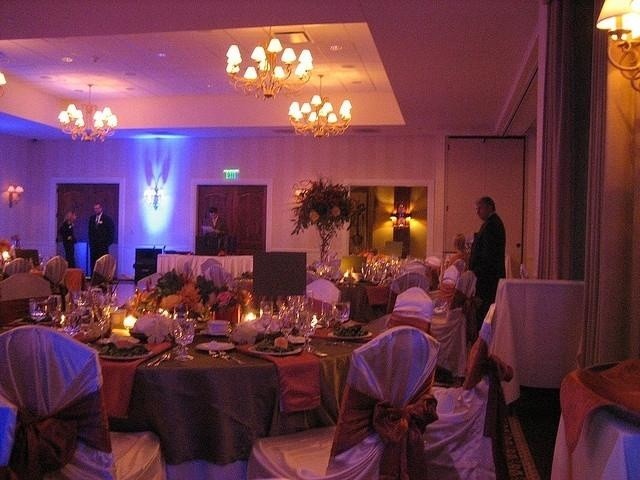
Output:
[
  {"xmin": 203, "ymin": 207, "xmax": 230, "ymax": 255},
  {"xmin": 58, "ymin": 211, "xmax": 77, "ymax": 268},
  {"xmin": 467, "ymin": 196, "xmax": 507, "ymax": 332},
  {"xmin": 443, "ymin": 233, "xmax": 467, "ymax": 273},
  {"xmin": 88, "ymin": 201, "xmax": 116, "ymax": 281}
]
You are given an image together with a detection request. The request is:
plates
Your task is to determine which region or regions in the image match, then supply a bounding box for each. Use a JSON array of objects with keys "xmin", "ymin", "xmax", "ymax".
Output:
[{"xmin": 328, "ymin": 330, "xmax": 373, "ymax": 339}]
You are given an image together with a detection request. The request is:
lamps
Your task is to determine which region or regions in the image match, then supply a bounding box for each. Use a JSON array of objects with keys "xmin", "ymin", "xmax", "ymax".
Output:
[
  {"xmin": 286, "ymin": 72, "xmax": 353, "ymax": 139},
  {"xmin": 145, "ymin": 176, "xmax": 167, "ymax": 209},
  {"xmin": 593, "ymin": 0, "xmax": 639, "ymax": 91},
  {"xmin": 221, "ymin": 26, "xmax": 315, "ymax": 102},
  {"xmin": 387, "ymin": 186, "xmax": 411, "ymax": 243},
  {"xmin": 2, "ymin": 184, "xmax": 25, "ymax": 209},
  {"xmin": 56, "ymin": 82, "xmax": 119, "ymax": 146}
]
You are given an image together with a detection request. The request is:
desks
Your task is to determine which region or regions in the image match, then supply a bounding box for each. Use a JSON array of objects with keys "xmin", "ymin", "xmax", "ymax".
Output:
[
  {"xmin": 548, "ymin": 361, "xmax": 639, "ymax": 479},
  {"xmin": 44, "ymin": 317, "xmax": 432, "ymax": 463}
]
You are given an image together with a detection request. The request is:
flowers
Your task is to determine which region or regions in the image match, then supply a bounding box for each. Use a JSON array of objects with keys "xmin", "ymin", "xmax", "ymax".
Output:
[{"xmin": 286, "ymin": 176, "xmax": 368, "ymax": 249}]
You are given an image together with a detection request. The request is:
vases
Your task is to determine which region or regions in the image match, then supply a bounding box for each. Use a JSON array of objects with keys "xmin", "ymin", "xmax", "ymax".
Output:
[{"xmin": 173, "ymin": 314, "xmax": 196, "ymax": 362}]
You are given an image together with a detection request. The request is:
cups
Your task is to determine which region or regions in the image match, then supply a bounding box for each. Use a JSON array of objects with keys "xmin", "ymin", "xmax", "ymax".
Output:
[{"xmin": 309, "ymin": 257, "xmax": 400, "ymax": 282}]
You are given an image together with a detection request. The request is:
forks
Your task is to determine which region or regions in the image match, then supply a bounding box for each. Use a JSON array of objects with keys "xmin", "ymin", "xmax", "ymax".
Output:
[{"xmin": 146, "ymin": 353, "xmax": 171, "ymax": 367}]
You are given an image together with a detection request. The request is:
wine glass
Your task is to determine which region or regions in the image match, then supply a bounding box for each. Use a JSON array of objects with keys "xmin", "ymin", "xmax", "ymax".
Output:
[{"xmin": 30, "ymin": 286, "xmax": 350, "ymax": 360}]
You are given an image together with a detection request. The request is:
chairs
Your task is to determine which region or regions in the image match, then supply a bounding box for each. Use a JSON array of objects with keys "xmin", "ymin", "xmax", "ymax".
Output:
[
  {"xmin": 1, "ymin": 325, "xmax": 167, "ymax": 477},
  {"xmin": 431, "ymin": 271, "xmax": 478, "ymax": 377},
  {"xmin": 245, "ymin": 325, "xmax": 442, "ymax": 477},
  {"xmin": 1, "ymin": 394, "xmax": 18, "ymax": 475},
  {"xmin": 130, "ymin": 248, "xmax": 477, "ymax": 328},
  {"xmin": 422, "ymin": 303, "xmax": 498, "ymax": 477},
  {"xmin": 491, "ymin": 277, "xmax": 586, "ymax": 409},
  {"xmin": 4, "ymin": 252, "xmax": 117, "ymax": 323}
]
